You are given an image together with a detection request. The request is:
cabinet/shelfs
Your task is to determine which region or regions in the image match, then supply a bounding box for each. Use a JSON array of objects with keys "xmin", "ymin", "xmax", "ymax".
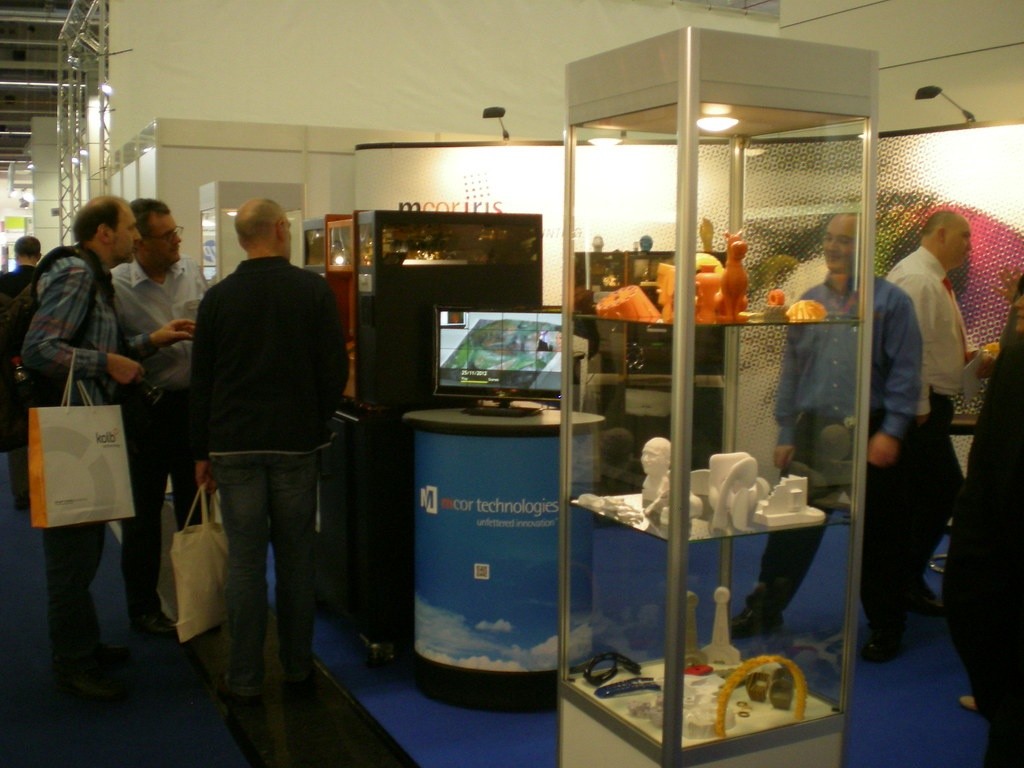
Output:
[{"xmin": 556, "ymin": 26, "xmax": 882, "ymax": 768}]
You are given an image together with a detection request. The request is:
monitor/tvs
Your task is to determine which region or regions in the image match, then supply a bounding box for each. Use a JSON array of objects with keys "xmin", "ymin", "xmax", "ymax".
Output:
[{"xmin": 434, "ymin": 306, "xmax": 564, "ymax": 416}]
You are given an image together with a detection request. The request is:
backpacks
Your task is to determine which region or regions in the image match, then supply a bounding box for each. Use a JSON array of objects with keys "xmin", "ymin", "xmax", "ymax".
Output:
[{"xmin": 0, "ymin": 248, "xmax": 95, "ymax": 453}]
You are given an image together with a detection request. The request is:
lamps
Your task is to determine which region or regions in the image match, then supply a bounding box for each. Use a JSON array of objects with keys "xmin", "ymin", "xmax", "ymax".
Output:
[
  {"xmin": 79, "ymin": 142, "xmax": 110, "ymax": 155},
  {"xmin": 24, "ymin": 159, "xmax": 34, "ymax": 169},
  {"xmin": 915, "ymin": 85, "xmax": 978, "ymax": 124},
  {"xmin": 67, "ymin": 48, "xmax": 133, "ymax": 72},
  {"xmin": 9, "ymin": 183, "xmax": 32, "ymax": 210},
  {"xmin": 72, "ymin": 151, "xmax": 79, "ymax": 163},
  {"xmin": 483, "ymin": 106, "xmax": 509, "ymax": 140}
]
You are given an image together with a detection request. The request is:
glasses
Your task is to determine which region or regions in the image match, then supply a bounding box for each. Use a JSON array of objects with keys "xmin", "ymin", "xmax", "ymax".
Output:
[{"xmin": 141, "ymin": 227, "xmax": 178, "ymax": 242}]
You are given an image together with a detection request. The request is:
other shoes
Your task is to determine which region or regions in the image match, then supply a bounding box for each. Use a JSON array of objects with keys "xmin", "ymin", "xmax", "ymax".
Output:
[
  {"xmin": 282, "ymin": 660, "xmax": 316, "ymax": 695},
  {"xmin": 131, "ymin": 612, "xmax": 179, "ymax": 634},
  {"xmin": 53, "ymin": 642, "xmax": 132, "ymax": 673},
  {"xmin": 959, "ymin": 694, "xmax": 979, "ymax": 712},
  {"xmin": 732, "ymin": 603, "xmax": 785, "ymax": 639},
  {"xmin": 13, "ymin": 503, "xmax": 30, "ymax": 510},
  {"xmin": 862, "ymin": 624, "xmax": 905, "ymax": 663},
  {"xmin": 907, "ymin": 589, "xmax": 946, "ymax": 618},
  {"xmin": 220, "ymin": 670, "xmax": 261, "ymax": 697}
]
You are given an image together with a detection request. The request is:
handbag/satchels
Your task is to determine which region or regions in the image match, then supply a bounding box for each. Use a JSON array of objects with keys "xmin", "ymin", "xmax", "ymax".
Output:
[
  {"xmin": 169, "ymin": 483, "xmax": 231, "ymax": 643},
  {"xmin": 27, "ymin": 349, "xmax": 136, "ymax": 529}
]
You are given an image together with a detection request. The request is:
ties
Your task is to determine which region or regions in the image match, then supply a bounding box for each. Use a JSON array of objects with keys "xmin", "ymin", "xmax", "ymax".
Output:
[{"xmin": 944, "ymin": 277, "xmax": 968, "ymax": 362}]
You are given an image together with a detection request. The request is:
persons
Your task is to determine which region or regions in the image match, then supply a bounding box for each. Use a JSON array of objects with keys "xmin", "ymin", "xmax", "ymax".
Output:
[
  {"xmin": 0, "ymin": 236, "xmax": 43, "ymax": 511},
  {"xmin": 884, "ymin": 211, "xmax": 977, "ymax": 616},
  {"xmin": 731, "ymin": 214, "xmax": 922, "ymax": 663},
  {"xmin": 22, "ymin": 196, "xmax": 196, "ymax": 703},
  {"xmin": 191, "ymin": 197, "xmax": 350, "ymax": 709},
  {"xmin": 640, "ymin": 437, "xmax": 670, "ymax": 490},
  {"xmin": 109, "ymin": 199, "xmax": 222, "ymax": 637},
  {"xmin": 536, "ymin": 331, "xmax": 562, "ymax": 351},
  {"xmin": 942, "ymin": 270, "xmax": 1024, "ymax": 768}
]
